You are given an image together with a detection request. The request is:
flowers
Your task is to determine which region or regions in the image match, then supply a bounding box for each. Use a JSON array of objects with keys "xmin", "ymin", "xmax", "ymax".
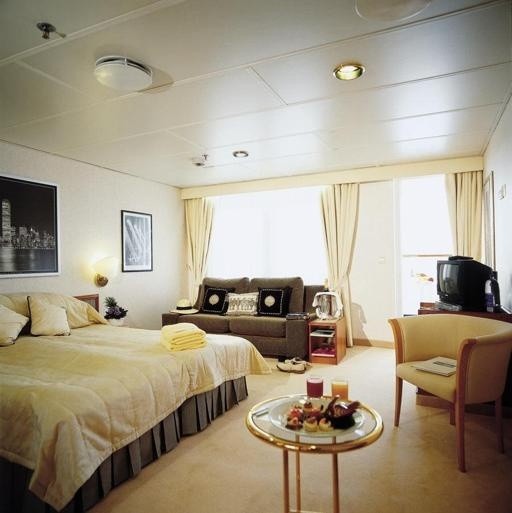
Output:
[{"xmin": 104, "ymin": 297, "xmax": 128, "ymax": 320}]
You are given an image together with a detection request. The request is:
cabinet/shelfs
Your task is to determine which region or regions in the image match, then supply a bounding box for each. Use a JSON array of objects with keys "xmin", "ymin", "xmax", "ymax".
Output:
[
  {"xmin": 416, "ymin": 302, "xmax": 512, "ymax": 419},
  {"xmin": 309, "ymin": 317, "xmax": 346, "ymax": 365}
]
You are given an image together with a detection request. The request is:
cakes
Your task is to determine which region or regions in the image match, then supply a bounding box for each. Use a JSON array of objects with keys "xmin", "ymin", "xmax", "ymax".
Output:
[{"xmin": 286, "ymin": 400, "xmax": 332, "ymax": 432}]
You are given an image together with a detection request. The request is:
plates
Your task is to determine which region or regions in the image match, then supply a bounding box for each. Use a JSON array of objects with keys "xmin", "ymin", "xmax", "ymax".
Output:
[{"xmin": 268, "ymin": 400, "xmax": 366, "ymax": 437}]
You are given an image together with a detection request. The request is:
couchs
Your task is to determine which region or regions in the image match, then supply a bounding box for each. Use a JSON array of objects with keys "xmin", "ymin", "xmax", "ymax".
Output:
[{"xmin": 162, "ymin": 277, "xmax": 324, "ymax": 359}]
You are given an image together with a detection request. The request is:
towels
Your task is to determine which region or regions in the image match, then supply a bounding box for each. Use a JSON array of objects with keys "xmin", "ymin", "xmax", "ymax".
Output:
[{"xmin": 162, "ymin": 323, "xmax": 206, "ymax": 351}]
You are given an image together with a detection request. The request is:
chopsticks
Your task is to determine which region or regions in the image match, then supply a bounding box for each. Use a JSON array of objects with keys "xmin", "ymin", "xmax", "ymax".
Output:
[{"xmin": 253, "ymin": 406, "xmax": 270, "ymax": 417}]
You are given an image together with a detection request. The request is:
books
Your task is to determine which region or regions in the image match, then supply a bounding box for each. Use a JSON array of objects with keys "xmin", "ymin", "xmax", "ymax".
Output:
[{"xmin": 415, "ymin": 354, "xmax": 457, "ymax": 377}]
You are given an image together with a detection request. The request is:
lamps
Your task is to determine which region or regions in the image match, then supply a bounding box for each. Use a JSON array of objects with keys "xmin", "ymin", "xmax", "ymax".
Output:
[
  {"xmin": 94, "ymin": 256, "xmax": 116, "ymax": 286},
  {"xmin": 36, "ymin": 23, "xmax": 65, "ymax": 39},
  {"xmin": 96, "ymin": 55, "xmax": 152, "ymax": 91},
  {"xmin": 193, "ymin": 154, "xmax": 208, "ymax": 166}
]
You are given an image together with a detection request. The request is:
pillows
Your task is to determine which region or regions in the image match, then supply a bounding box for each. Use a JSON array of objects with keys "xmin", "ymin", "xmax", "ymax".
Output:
[
  {"xmin": 227, "ymin": 292, "xmax": 260, "ymax": 315},
  {"xmin": 258, "ymin": 286, "xmax": 292, "ymax": 316},
  {"xmin": 0, "ymin": 293, "xmax": 107, "ymax": 328},
  {"xmin": 0, "ymin": 305, "xmax": 29, "ymax": 346},
  {"xmin": 200, "ymin": 285, "xmax": 235, "ymax": 315},
  {"xmin": 26, "ymin": 297, "xmax": 70, "ymax": 336}
]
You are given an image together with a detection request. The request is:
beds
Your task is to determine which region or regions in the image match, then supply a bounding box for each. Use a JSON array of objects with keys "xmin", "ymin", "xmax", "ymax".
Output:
[{"xmin": 0, "ymin": 295, "xmax": 271, "ymax": 513}]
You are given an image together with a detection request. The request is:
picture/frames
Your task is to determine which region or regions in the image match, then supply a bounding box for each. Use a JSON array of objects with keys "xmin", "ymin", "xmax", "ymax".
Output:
[
  {"xmin": 482, "ymin": 171, "xmax": 495, "ymax": 291},
  {"xmin": 121, "ymin": 210, "xmax": 153, "ymax": 272},
  {"xmin": 0, "ymin": 175, "xmax": 61, "ymax": 279}
]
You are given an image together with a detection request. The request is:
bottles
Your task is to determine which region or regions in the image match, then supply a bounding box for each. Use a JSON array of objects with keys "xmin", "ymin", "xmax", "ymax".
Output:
[
  {"xmin": 315, "ymin": 293, "xmax": 341, "ymax": 320},
  {"xmin": 484, "ymin": 272, "xmax": 501, "ymax": 313}
]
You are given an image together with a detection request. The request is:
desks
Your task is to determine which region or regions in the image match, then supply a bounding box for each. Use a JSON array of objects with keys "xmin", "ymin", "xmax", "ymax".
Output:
[{"xmin": 247, "ymin": 394, "xmax": 383, "ymax": 513}]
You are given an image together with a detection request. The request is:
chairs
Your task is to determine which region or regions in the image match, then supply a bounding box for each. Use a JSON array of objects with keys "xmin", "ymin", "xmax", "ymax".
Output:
[{"xmin": 388, "ymin": 313, "xmax": 512, "ymax": 472}]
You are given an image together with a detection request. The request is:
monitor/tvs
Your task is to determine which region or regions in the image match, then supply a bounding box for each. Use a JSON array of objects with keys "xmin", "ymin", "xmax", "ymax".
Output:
[{"xmin": 437, "ymin": 260, "xmax": 492, "ymax": 312}]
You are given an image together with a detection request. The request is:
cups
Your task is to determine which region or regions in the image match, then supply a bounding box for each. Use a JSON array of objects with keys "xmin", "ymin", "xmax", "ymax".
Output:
[
  {"xmin": 331, "ymin": 378, "xmax": 349, "ymax": 402},
  {"xmin": 306, "ymin": 376, "xmax": 324, "ymax": 398}
]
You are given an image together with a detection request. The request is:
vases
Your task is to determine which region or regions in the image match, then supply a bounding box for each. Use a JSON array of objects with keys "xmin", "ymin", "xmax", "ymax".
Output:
[{"xmin": 110, "ymin": 319, "xmax": 124, "ymax": 326}]
